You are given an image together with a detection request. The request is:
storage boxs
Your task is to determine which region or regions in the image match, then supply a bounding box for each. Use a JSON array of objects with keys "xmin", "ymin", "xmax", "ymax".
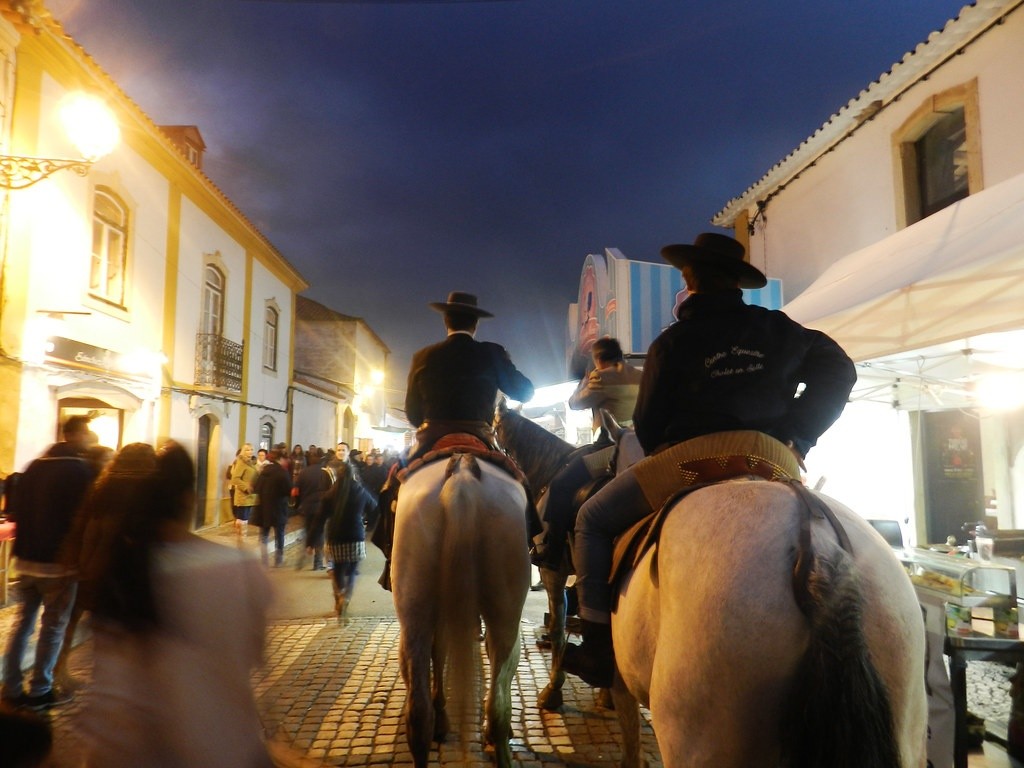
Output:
[{"xmin": 972, "ymin": 607, "xmax": 1016, "ymax": 636}]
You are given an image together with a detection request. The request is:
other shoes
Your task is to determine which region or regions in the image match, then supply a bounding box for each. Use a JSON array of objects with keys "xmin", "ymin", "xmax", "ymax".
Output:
[
  {"xmin": 341, "ymin": 601, "xmax": 349, "ymax": 614},
  {"xmin": 541, "ymin": 634, "xmax": 551, "ymax": 641},
  {"xmin": 3, "ymin": 692, "xmax": 28, "ymax": 708},
  {"xmin": 29, "ymin": 687, "xmax": 75, "ymax": 711},
  {"xmin": 536, "ymin": 641, "xmax": 552, "ymax": 649},
  {"xmin": 335, "ymin": 595, "xmax": 344, "ymax": 610},
  {"xmin": 531, "ymin": 549, "xmax": 559, "ymax": 571}
]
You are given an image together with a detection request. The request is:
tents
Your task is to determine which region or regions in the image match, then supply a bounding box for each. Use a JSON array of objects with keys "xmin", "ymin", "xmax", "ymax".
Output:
[{"xmin": 778, "ymin": 174, "xmax": 1024, "ymax": 552}]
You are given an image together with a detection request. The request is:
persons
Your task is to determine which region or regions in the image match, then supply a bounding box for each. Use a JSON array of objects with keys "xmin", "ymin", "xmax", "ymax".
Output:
[
  {"xmin": 2, "ymin": 412, "xmax": 119, "ymax": 711},
  {"xmin": 307, "ymin": 462, "xmax": 379, "ymax": 627},
  {"xmin": 531, "ymin": 337, "xmax": 645, "ymax": 572},
  {"xmin": 225, "ymin": 441, "xmax": 420, "ymax": 573},
  {"xmin": 373, "ymin": 290, "xmax": 540, "ymax": 594},
  {"xmin": 72, "ymin": 438, "xmax": 279, "ymax": 768},
  {"xmin": 559, "ymin": 231, "xmax": 858, "ymax": 690}
]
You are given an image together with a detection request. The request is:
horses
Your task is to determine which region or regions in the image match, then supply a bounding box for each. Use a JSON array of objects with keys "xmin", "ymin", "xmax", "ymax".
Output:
[
  {"xmin": 606, "ymin": 473, "xmax": 930, "ymax": 768},
  {"xmin": 388, "ymin": 414, "xmax": 534, "ymax": 767},
  {"xmin": 490, "ymin": 394, "xmax": 625, "ymax": 715}
]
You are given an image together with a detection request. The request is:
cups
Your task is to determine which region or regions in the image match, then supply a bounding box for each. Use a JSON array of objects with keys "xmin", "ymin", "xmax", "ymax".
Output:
[
  {"xmin": 967, "ymin": 539, "xmax": 978, "ymax": 552},
  {"xmin": 974, "ymin": 537, "xmax": 994, "ymax": 565}
]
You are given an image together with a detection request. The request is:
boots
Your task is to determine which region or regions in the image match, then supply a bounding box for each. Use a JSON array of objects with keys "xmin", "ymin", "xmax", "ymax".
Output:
[{"xmin": 562, "ymin": 618, "xmax": 615, "ymax": 688}]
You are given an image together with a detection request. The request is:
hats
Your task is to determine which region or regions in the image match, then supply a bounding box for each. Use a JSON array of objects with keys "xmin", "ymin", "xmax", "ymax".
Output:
[
  {"xmin": 427, "ymin": 291, "xmax": 494, "ymax": 318},
  {"xmin": 660, "ymin": 233, "xmax": 767, "ymax": 289},
  {"xmin": 349, "ymin": 449, "xmax": 363, "ymax": 456}
]
